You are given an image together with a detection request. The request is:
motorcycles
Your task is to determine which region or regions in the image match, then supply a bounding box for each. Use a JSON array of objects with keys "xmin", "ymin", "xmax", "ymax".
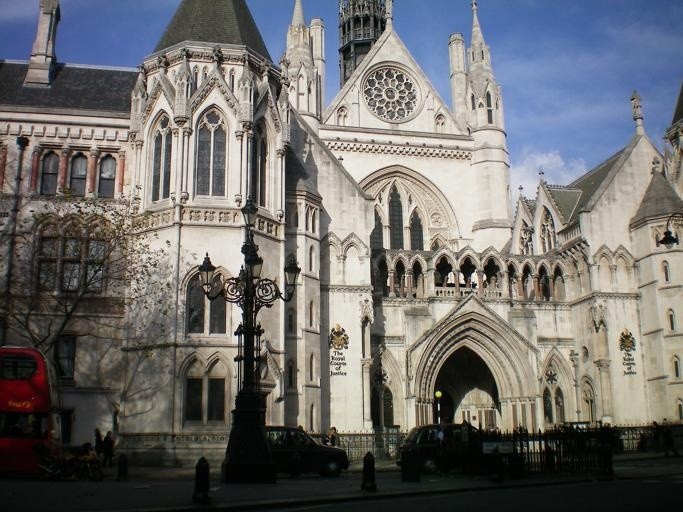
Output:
[{"xmin": 33, "ymin": 442, "xmax": 102, "ymax": 480}]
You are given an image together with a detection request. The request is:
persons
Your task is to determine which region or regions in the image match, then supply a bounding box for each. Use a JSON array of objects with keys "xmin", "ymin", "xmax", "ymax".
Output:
[
  {"xmin": 326, "ymin": 426, "xmax": 340, "ymax": 445},
  {"xmin": 487, "ymin": 420, "xmax": 682, "ymax": 484},
  {"xmin": 102, "ymin": 428, "xmax": 117, "ymax": 467},
  {"xmin": 95, "ymin": 426, "xmax": 103, "ymax": 456}
]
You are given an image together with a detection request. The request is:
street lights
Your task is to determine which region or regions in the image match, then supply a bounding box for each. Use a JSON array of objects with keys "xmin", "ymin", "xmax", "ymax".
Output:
[{"xmin": 197, "ymin": 191, "xmax": 302, "ymax": 485}]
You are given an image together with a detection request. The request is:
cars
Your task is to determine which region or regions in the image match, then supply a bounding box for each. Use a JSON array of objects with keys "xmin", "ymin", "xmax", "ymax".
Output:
[
  {"xmin": 265, "ymin": 426, "xmax": 349, "ymax": 478},
  {"xmin": 397, "ymin": 423, "xmax": 480, "ymax": 475}
]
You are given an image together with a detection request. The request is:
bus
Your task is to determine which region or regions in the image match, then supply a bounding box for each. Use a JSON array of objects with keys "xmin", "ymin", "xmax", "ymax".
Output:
[{"xmin": 0, "ymin": 346, "xmax": 62, "ymax": 476}]
